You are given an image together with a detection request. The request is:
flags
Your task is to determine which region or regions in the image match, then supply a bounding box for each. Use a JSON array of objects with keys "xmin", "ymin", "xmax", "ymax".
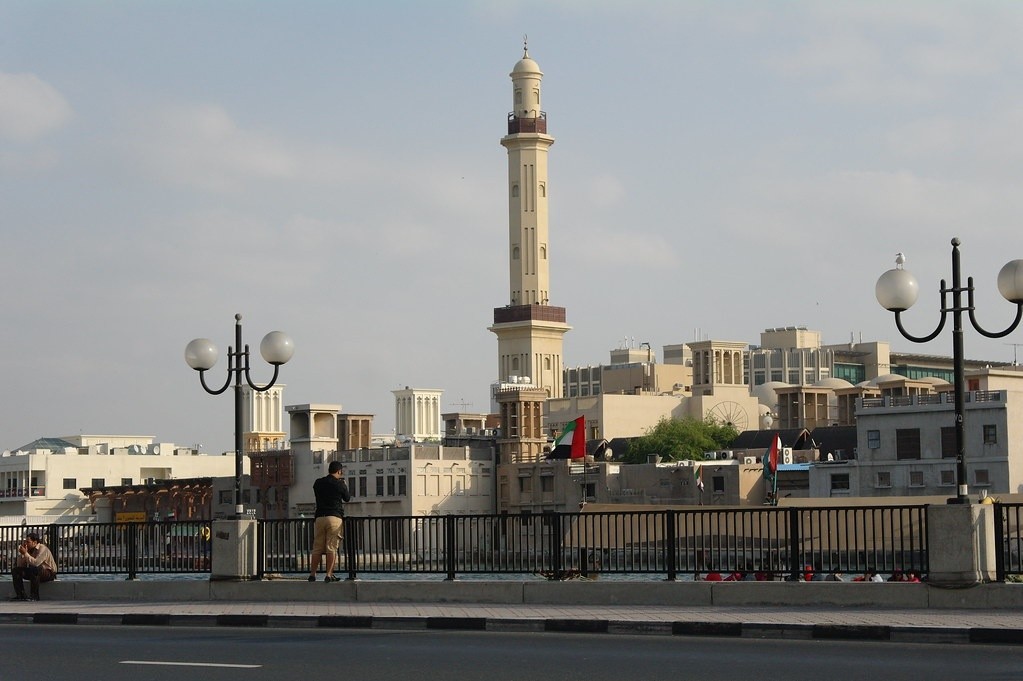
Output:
[
  {"xmin": 695, "ymin": 465, "xmax": 704, "ymax": 493},
  {"xmin": 763, "ymin": 435, "xmax": 782, "ymax": 481},
  {"xmin": 540, "ymin": 416, "xmax": 585, "ymax": 460}
]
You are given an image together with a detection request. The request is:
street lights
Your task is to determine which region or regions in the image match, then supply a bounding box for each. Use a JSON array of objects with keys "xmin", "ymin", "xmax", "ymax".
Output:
[
  {"xmin": 873, "ymin": 237, "xmax": 1023, "ymax": 505},
  {"xmin": 184, "ymin": 310, "xmax": 296, "ymax": 520}
]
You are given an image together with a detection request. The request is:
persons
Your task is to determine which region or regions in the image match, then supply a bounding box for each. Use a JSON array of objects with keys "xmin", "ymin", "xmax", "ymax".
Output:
[
  {"xmin": 81, "ymin": 540, "xmax": 88, "ymax": 557},
  {"xmin": 10, "ymin": 533, "xmax": 58, "ymax": 601},
  {"xmin": 308, "ymin": 461, "xmax": 350, "ymax": 583},
  {"xmin": 978, "ymin": 489, "xmax": 992, "ymax": 505},
  {"xmin": 166, "ymin": 531, "xmax": 171, "ymax": 556},
  {"xmin": 703, "ymin": 562, "xmax": 918, "ymax": 581}
]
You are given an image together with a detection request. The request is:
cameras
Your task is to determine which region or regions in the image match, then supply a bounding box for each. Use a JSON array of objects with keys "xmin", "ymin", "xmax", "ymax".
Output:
[{"xmin": 341, "ymin": 478, "xmax": 345, "ymax": 483}]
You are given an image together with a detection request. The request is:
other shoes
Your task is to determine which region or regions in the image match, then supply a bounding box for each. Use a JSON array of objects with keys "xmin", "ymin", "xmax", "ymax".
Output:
[
  {"xmin": 324, "ymin": 575, "xmax": 341, "ymax": 582},
  {"xmin": 307, "ymin": 575, "xmax": 316, "ymax": 581},
  {"xmin": 10, "ymin": 595, "xmax": 26, "ymax": 600},
  {"xmin": 26, "ymin": 596, "xmax": 40, "ymax": 602}
]
